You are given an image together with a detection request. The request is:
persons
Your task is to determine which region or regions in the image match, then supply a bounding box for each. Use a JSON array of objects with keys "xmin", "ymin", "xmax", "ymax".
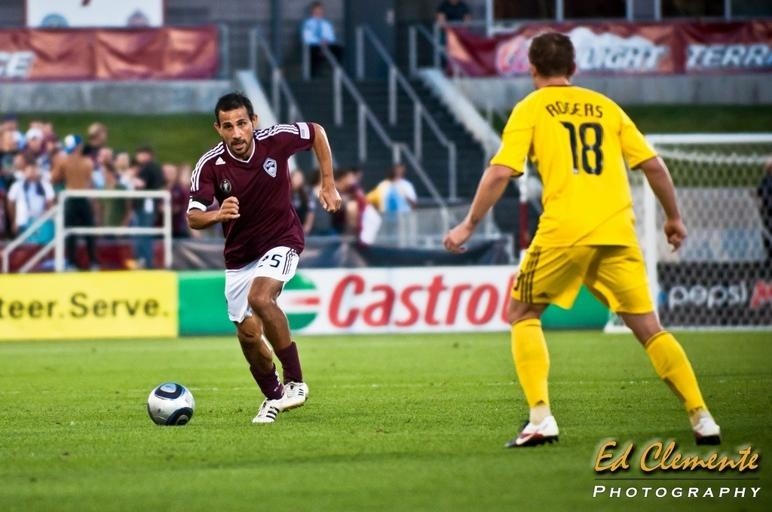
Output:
[
  {"xmin": 300, "ymin": 2, "xmax": 346, "ymax": 84},
  {"xmin": 185, "ymin": 92, "xmax": 343, "ymax": 426},
  {"xmin": 0, "ymin": 108, "xmax": 420, "ymax": 268},
  {"xmin": 496, "ymin": 24, "xmax": 534, "ymax": 73},
  {"xmin": 749, "ymin": 155, "xmax": 771, "ymax": 275},
  {"xmin": 433, "ymin": 0, "xmax": 471, "ymax": 29},
  {"xmin": 443, "ymin": 31, "xmax": 725, "ymax": 448}
]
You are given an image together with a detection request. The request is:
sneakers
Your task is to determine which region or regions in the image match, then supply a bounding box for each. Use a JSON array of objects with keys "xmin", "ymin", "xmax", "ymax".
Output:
[
  {"xmin": 693, "ymin": 418, "xmax": 722, "ymax": 445},
  {"xmin": 279, "ymin": 381, "xmax": 308, "ymax": 409},
  {"xmin": 252, "ymin": 398, "xmax": 283, "ymax": 423},
  {"xmin": 504, "ymin": 416, "xmax": 559, "ymax": 448}
]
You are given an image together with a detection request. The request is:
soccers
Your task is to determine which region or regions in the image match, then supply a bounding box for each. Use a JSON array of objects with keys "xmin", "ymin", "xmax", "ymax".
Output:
[{"xmin": 147, "ymin": 383, "xmax": 195, "ymax": 425}]
